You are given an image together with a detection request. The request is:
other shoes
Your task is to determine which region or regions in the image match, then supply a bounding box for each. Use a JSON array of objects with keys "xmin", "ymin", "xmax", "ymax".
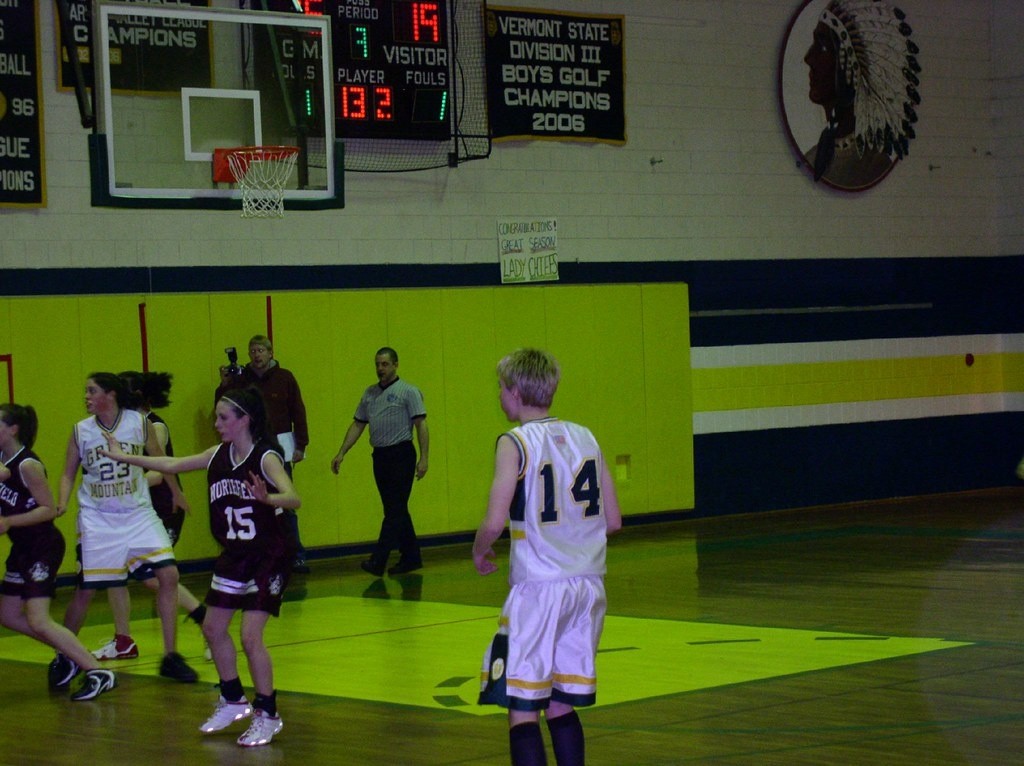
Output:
[
  {"xmin": 388, "ymin": 557, "xmax": 423, "ymax": 574},
  {"xmin": 293, "ymin": 558, "xmax": 310, "ymax": 572},
  {"xmin": 361, "ymin": 559, "xmax": 384, "ymax": 576}
]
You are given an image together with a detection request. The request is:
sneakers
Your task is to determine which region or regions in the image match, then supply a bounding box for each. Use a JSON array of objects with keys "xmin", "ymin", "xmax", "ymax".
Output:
[
  {"xmin": 90, "ymin": 639, "xmax": 138, "ymax": 660},
  {"xmin": 236, "ymin": 709, "xmax": 283, "ymax": 747},
  {"xmin": 198, "ymin": 694, "xmax": 254, "ymax": 733},
  {"xmin": 204, "ymin": 644, "xmax": 213, "ymax": 661},
  {"xmin": 70, "ymin": 669, "xmax": 119, "ymax": 701},
  {"xmin": 49, "ymin": 659, "xmax": 71, "ymax": 693},
  {"xmin": 160, "ymin": 653, "xmax": 198, "ymax": 682},
  {"xmin": 54, "ymin": 656, "xmax": 82, "ymax": 686}
]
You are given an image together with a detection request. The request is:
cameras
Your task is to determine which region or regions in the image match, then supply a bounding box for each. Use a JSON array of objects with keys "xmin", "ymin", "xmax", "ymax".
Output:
[{"xmin": 222, "ymin": 347, "xmax": 240, "ymax": 377}]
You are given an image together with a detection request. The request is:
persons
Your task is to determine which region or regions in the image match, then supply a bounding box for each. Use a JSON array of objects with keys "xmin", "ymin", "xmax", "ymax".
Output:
[
  {"xmin": 91, "ymin": 370, "xmax": 212, "ymax": 663},
  {"xmin": 57, "ymin": 372, "xmax": 201, "ymax": 681},
  {"xmin": 473, "ymin": 348, "xmax": 622, "ymax": 766},
  {"xmin": 330, "ymin": 347, "xmax": 429, "ymax": 576},
  {"xmin": 0, "ymin": 403, "xmax": 118, "ymax": 702},
  {"xmin": 215, "ymin": 335, "xmax": 309, "ymax": 574},
  {"xmin": 97, "ymin": 388, "xmax": 302, "ymax": 746}
]
